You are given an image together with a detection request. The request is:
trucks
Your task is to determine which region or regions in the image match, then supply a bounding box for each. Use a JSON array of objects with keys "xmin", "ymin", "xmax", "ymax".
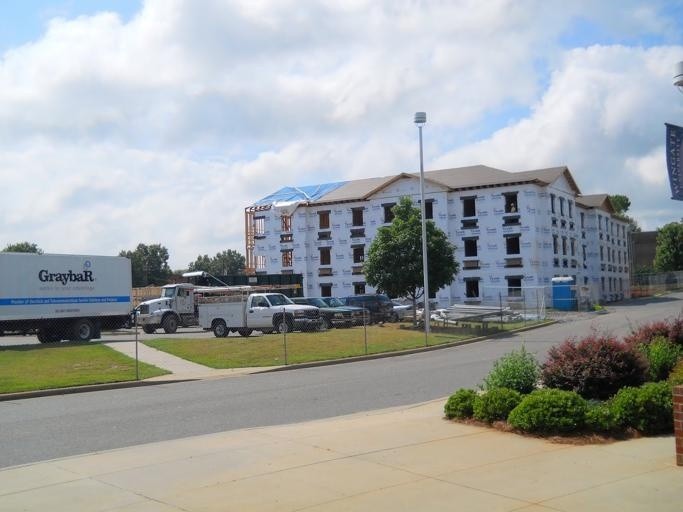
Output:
[
  {"xmin": 195, "ymin": 291, "xmax": 320, "ymax": 338},
  {"xmin": 135, "ymin": 281, "xmax": 260, "ymax": 334}
]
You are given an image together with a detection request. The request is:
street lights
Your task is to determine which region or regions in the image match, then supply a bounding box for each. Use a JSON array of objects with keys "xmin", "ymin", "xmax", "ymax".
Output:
[{"xmin": 411, "ymin": 111, "xmax": 434, "ymax": 334}]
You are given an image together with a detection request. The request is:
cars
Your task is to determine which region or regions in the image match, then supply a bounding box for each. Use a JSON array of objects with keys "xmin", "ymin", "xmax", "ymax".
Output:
[
  {"xmin": 287, "ymin": 297, "xmax": 371, "ymax": 332},
  {"xmin": 385, "ymin": 299, "xmax": 415, "ymax": 324}
]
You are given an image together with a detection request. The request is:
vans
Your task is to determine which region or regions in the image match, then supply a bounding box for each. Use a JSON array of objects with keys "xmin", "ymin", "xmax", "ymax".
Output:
[{"xmin": 336, "ymin": 296, "xmax": 393, "ymax": 325}]
